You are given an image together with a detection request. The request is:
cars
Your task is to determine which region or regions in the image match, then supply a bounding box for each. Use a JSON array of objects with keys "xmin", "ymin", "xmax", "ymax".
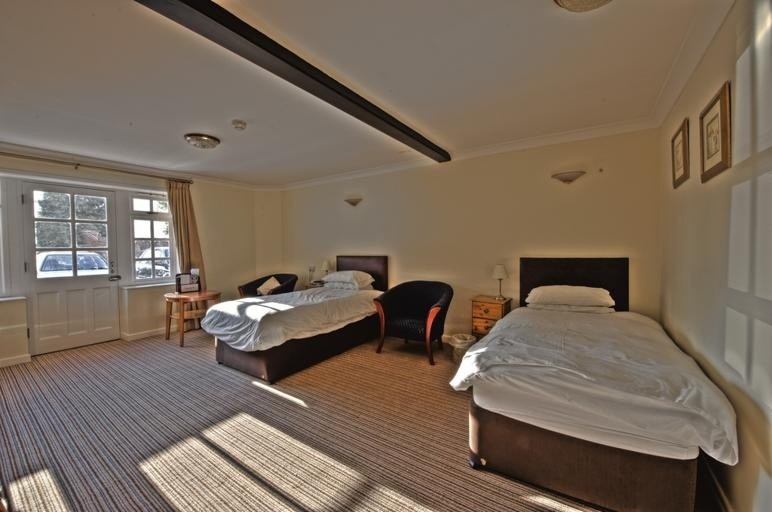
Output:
[
  {"xmin": 34, "ymin": 249, "xmax": 108, "ymax": 278},
  {"xmin": 137, "ymin": 245, "xmax": 170, "ymax": 278}
]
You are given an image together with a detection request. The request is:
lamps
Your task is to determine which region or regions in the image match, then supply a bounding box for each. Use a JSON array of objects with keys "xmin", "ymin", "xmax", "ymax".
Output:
[
  {"xmin": 345, "ymin": 197, "xmax": 362, "ymax": 205},
  {"xmin": 491, "ymin": 264, "xmax": 508, "ymax": 299},
  {"xmin": 556, "ymin": 1, "xmax": 612, "ymax": 14},
  {"xmin": 185, "ymin": 134, "xmax": 220, "ymax": 149},
  {"xmin": 320, "ymin": 259, "xmax": 332, "ymax": 285},
  {"xmin": 550, "ymin": 170, "xmax": 584, "ymax": 184}
]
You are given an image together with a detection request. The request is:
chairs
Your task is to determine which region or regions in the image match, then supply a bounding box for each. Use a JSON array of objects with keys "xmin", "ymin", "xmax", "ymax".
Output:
[
  {"xmin": 373, "ymin": 280, "xmax": 453, "ymax": 364},
  {"xmin": 237, "ymin": 274, "xmax": 297, "ymax": 298}
]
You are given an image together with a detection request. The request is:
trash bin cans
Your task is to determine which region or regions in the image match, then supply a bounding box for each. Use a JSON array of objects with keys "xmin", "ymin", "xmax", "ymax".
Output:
[{"xmin": 453, "ymin": 334, "xmax": 477, "ymax": 364}]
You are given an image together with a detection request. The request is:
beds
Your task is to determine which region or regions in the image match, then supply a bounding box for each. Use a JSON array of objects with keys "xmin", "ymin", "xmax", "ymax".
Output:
[
  {"xmin": 200, "ymin": 255, "xmax": 387, "ymax": 383},
  {"xmin": 450, "ymin": 256, "xmax": 740, "ymax": 511}
]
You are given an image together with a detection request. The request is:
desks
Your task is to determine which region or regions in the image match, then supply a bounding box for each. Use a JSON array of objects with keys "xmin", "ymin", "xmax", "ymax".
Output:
[{"xmin": 164, "ymin": 288, "xmax": 222, "ymax": 347}]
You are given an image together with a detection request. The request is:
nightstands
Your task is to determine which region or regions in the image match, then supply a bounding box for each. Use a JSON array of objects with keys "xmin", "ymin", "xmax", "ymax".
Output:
[
  {"xmin": 303, "ymin": 281, "xmax": 326, "ymax": 289},
  {"xmin": 470, "ymin": 295, "xmax": 512, "ymax": 343}
]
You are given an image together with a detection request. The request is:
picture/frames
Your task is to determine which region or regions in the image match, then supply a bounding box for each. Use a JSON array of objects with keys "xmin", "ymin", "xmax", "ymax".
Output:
[{"xmin": 670, "ymin": 79, "xmax": 731, "ymax": 189}]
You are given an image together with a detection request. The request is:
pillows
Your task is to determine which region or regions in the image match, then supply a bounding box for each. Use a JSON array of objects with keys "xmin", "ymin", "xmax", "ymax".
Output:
[
  {"xmin": 321, "ymin": 270, "xmax": 375, "ymax": 291},
  {"xmin": 524, "ymin": 284, "xmax": 616, "ymax": 312},
  {"xmin": 258, "ymin": 277, "xmax": 281, "ymax": 294}
]
